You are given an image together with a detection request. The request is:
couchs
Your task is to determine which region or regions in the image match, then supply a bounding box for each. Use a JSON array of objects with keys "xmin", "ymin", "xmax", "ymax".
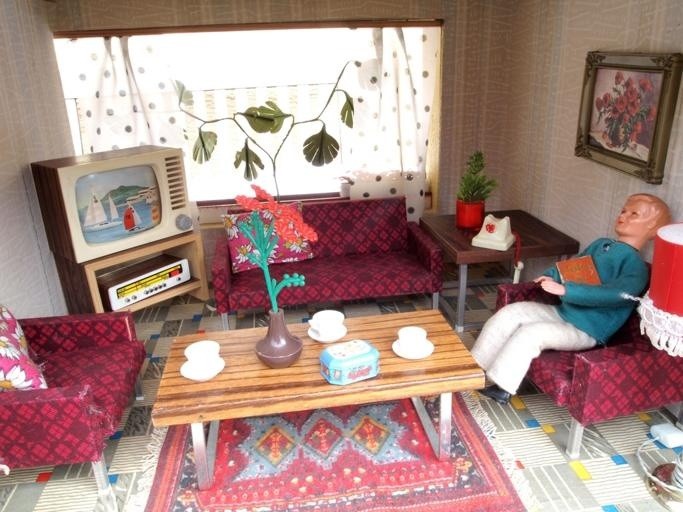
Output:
[
  {"xmin": 495, "ymin": 277, "xmax": 680, "ymax": 456},
  {"xmin": 209, "ymin": 194, "xmax": 444, "ymax": 328},
  {"xmin": 0, "ymin": 310, "xmax": 146, "ymax": 512}
]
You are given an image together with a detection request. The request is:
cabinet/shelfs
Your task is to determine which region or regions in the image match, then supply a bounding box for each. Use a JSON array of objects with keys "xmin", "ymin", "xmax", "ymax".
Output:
[{"xmin": 56, "ymin": 233, "xmax": 209, "ymax": 320}]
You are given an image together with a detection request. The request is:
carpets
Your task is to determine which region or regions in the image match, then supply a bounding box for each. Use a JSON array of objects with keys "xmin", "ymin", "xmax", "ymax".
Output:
[{"xmin": 131, "ymin": 392, "xmax": 537, "ymax": 512}]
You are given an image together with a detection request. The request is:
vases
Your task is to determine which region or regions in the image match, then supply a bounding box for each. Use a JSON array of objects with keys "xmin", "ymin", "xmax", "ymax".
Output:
[{"xmin": 253, "ymin": 307, "xmax": 303, "ymax": 368}]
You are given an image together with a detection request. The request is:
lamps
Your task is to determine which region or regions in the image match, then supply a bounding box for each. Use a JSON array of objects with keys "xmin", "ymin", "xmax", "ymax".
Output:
[{"xmin": 638, "ymin": 221, "xmax": 681, "ymax": 448}]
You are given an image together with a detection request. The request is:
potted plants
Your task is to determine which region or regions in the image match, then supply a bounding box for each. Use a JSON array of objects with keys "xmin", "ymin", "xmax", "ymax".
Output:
[{"xmin": 453, "ymin": 150, "xmax": 497, "ymax": 229}]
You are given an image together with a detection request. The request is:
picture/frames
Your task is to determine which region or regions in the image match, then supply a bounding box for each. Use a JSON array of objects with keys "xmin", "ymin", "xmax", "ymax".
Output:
[{"xmin": 571, "ymin": 48, "xmax": 681, "ymax": 185}]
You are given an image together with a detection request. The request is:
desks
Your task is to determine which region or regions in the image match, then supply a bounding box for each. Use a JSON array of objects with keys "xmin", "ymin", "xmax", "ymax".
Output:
[{"xmin": 418, "ymin": 209, "xmax": 579, "ymax": 332}]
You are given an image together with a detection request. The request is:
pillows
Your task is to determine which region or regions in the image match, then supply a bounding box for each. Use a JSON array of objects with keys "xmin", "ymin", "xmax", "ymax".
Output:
[
  {"xmin": 0, "ymin": 303, "xmax": 47, "ymax": 391},
  {"xmin": 221, "ymin": 203, "xmax": 313, "ymax": 268}
]
